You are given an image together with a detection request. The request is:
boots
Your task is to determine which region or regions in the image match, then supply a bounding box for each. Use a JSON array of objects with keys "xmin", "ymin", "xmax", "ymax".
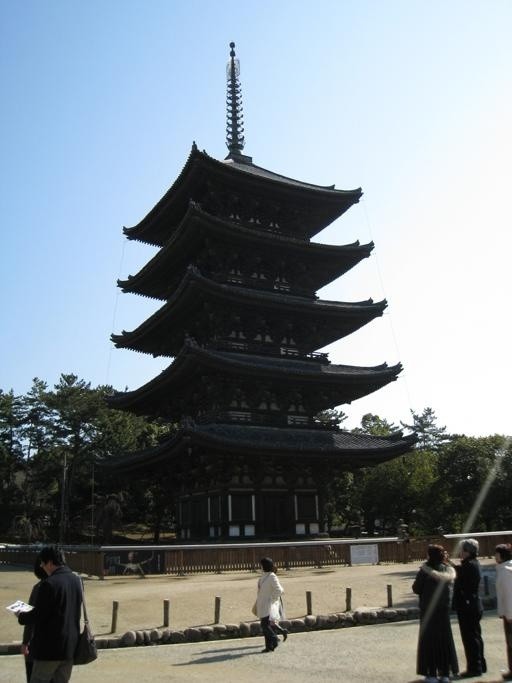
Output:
[{"xmin": 271, "ymin": 624, "xmax": 290, "ymax": 642}]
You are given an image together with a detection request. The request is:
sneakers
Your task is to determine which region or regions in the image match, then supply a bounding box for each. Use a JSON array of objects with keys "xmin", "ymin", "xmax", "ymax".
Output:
[
  {"xmin": 419, "ymin": 663, "xmax": 511, "ymax": 683},
  {"xmin": 260, "ymin": 638, "xmax": 282, "ymax": 654}
]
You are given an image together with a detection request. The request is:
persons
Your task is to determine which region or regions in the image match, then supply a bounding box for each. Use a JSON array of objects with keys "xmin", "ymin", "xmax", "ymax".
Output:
[
  {"xmin": 411, "ymin": 543, "xmax": 460, "ymax": 683},
  {"xmin": 445, "ymin": 537, "xmax": 488, "ymax": 676},
  {"xmin": 493, "ymin": 542, "xmax": 511, "ymax": 682},
  {"xmin": 254, "ymin": 555, "xmax": 285, "ymax": 653},
  {"xmin": 18, "ymin": 552, "xmax": 48, "ymax": 682},
  {"xmin": 119, "ymin": 551, "xmax": 146, "ymax": 575},
  {"xmin": 269, "ymin": 565, "xmax": 289, "ymax": 642},
  {"xmin": 11, "ymin": 544, "xmax": 85, "ymax": 682}
]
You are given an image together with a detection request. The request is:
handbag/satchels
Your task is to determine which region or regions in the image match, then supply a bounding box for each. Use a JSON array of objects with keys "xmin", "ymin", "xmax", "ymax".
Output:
[{"xmin": 72, "ymin": 575, "xmax": 99, "ymax": 666}]
